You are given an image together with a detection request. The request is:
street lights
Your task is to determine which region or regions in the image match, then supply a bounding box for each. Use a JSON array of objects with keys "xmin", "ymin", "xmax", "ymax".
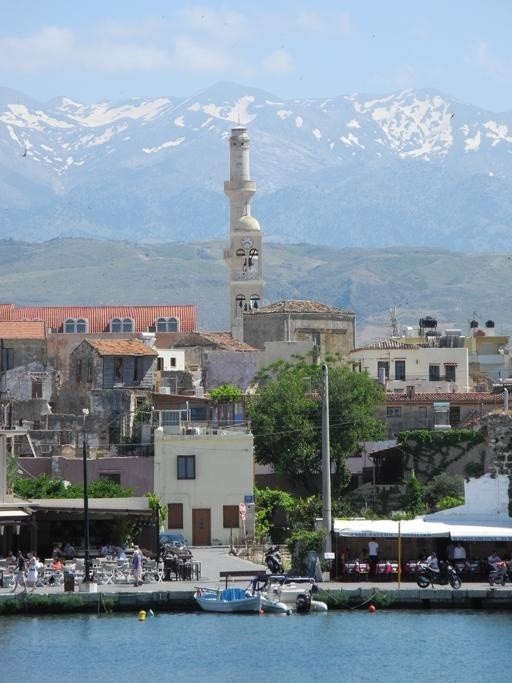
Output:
[{"xmin": 81, "ymin": 407, "xmax": 94, "ymax": 582}]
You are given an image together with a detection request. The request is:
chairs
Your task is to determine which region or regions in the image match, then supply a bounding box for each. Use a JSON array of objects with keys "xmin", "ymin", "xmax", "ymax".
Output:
[
  {"xmin": 343, "ymin": 557, "xmax": 511, "ymax": 582},
  {"xmin": 0, "ymin": 556, "xmax": 203, "ymax": 585}
]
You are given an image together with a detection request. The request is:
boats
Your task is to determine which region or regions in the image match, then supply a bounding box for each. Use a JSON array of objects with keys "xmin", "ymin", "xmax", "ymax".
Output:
[
  {"xmin": 190, "ymin": 585, "xmax": 263, "ymax": 611},
  {"xmin": 244, "ymin": 572, "xmax": 329, "ymax": 615}
]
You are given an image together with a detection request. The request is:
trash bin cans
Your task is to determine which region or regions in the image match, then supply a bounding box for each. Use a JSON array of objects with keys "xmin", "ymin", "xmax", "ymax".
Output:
[{"xmin": 64, "ymin": 574, "xmax": 74, "ymax": 592}]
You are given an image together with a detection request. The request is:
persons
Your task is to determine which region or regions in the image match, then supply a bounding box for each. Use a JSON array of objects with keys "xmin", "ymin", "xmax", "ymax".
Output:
[
  {"xmin": 100, "ymin": 542, "xmax": 127, "ymax": 565},
  {"xmin": 338, "ymin": 551, "xmax": 346, "ymax": 583},
  {"xmin": 265, "ymin": 544, "xmax": 284, "ymax": 574},
  {"xmin": 154, "ymin": 542, "xmax": 192, "ymax": 580},
  {"xmin": 123, "ymin": 536, "xmax": 154, "ymax": 587},
  {"xmin": 368, "ymin": 537, "xmax": 379, "ymax": 576},
  {"xmin": 350, "ymin": 560, "xmax": 424, "ymax": 582},
  {"xmin": 443, "ymin": 541, "xmax": 467, "ymax": 562},
  {"xmin": 416, "ymin": 550, "xmax": 439, "ymax": 570},
  {"xmin": 229, "ymin": 544, "xmax": 245, "ymax": 556},
  {"xmin": 52, "ymin": 540, "xmax": 76, "ymax": 570},
  {"xmin": 488, "ymin": 550, "xmax": 510, "ymax": 582},
  {"xmin": 6, "ymin": 550, "xmax": 39, "ymax": 593}
]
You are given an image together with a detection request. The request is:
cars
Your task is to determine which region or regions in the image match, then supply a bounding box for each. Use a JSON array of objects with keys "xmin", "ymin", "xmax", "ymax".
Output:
[{"xmin": 158, "ymin": 532, "xmax": 188, "ymax": 550}]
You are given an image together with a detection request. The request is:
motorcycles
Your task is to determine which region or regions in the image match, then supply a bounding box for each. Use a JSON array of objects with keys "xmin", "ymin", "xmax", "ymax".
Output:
[
  {"xmin": 415, "ymin": 561, "xmax": 461, "ymax": 588},
  {"xmin": 264, "ymin": 544, "xmax": 285, "ymax": 573},
  {"xmin": 488, "ymin": 560, "xmax": 507, "ymax": 587}
]
